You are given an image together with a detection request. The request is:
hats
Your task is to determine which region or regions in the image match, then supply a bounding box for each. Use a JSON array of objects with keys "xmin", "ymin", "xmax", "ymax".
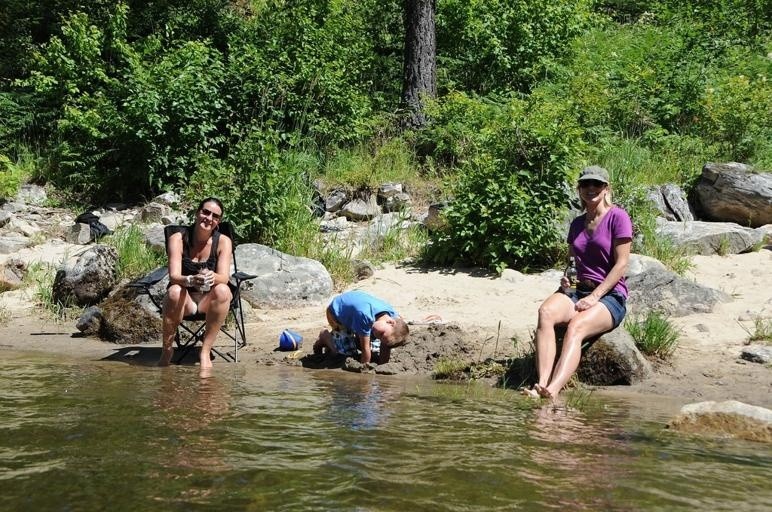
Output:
[{"xmin": 578, "ymin": 165, "xmax": 609, "ymax": 185}]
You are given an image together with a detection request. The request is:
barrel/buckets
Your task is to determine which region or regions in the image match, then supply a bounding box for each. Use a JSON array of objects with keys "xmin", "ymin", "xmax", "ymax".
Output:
[{"xmin": 279, "ymin": 328, "xmax": 302, "ymax": 350}]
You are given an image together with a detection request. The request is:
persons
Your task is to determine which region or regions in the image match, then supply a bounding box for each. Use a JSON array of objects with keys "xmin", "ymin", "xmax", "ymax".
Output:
[
  {"xmin": 313, "ymin": 289, "xmax": 409, "ymax": 365},
  {"xmin": 520, "ymin": 164, "xmax": 634, "ymax": 399},
  {"xmin": 158, "ymin": 198, "xmax": 233, "ymax": 377}
]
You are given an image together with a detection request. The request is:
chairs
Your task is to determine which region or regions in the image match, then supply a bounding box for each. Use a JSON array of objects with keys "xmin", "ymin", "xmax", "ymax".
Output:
[{"xmin": 164, "ymin": 219, "xmax": 259, "ymax": 362}]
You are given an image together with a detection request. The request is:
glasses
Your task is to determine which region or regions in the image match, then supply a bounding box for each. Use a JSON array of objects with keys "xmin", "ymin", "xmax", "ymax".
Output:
[
  {"xmin": 198, "ymin": 207, "xmax": 221, "ymax": 219},
  {"xmin": 580, "ymin": 179, "xmax": 603, "ymax": 189}
]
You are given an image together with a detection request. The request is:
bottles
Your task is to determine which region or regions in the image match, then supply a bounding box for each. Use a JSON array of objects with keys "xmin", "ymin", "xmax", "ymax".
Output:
[
  {"xmin": 565, "ymin": 256, "xmax": 576, "ymax": 292},
  {"xmin": 200, "ymin": 263, "xmax": 211, "ymax": 291}
]
